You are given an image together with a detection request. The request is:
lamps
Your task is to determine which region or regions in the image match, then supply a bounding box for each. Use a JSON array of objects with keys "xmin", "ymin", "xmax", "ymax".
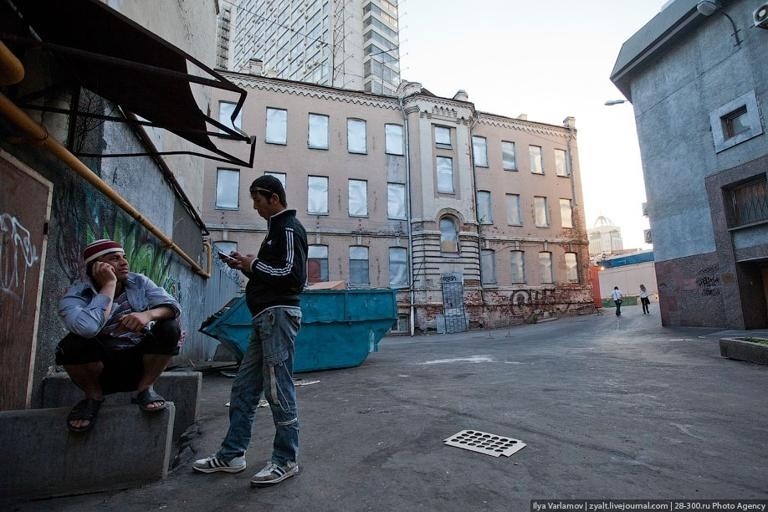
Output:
[
  {"xmin": 697, "ymin": 0, "xmax": 743, "ymax": 47},
  {"xmin": 604, "ymin": 99, "xmax": 628, "ymax": 105}
]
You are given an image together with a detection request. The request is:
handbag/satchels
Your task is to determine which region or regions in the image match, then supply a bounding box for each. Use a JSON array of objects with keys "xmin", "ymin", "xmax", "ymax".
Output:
[{"xmin": 617, "ymin": 299, "xmax": 623, "ymax": 304}]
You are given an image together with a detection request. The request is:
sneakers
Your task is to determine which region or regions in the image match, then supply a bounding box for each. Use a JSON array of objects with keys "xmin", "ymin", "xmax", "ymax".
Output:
[
  {"xmin": 192, "ymin": 455, "xmax": 247, "ymax": 473},
  {"xmin": 249, "ymin": 457, "xmax": 299, "ymax": 484}
]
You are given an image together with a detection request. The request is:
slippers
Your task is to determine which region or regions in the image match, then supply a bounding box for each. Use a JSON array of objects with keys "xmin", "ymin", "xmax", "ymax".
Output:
[
  {"xmin": 137, "ymin": 388, "xmax": 167, "ymax": 412},
  {"xmin": 64, "ymin": 398, "xmax": 104, "ymax": 435}
]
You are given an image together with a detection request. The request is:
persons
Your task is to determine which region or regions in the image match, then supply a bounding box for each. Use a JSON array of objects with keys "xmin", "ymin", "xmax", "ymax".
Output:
[
  {"xmin": 639, "ymin": 284, "xmax": 650, "ymax": 315},
  {"xmin": 190, "ymin": 174, "xmax": 309, "ymax": 489},
  {"xmin": 54, "ymin": 238, "xmax": 183, "ymax": 433},
  {"xmin": 610, "ymin": 286, "xmax": 624, "ymax": 317}
]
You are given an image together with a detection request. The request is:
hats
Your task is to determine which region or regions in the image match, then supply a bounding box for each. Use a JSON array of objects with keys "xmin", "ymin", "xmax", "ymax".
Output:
[{"xmin": 81, "ymin": 239, "xmax": 124, "ymax": 264}]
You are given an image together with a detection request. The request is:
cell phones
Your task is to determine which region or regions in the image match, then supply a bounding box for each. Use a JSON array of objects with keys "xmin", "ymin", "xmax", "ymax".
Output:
[{"xmin": 218, "ymin": 251, "xmax": 237, "ymax": 262}]
